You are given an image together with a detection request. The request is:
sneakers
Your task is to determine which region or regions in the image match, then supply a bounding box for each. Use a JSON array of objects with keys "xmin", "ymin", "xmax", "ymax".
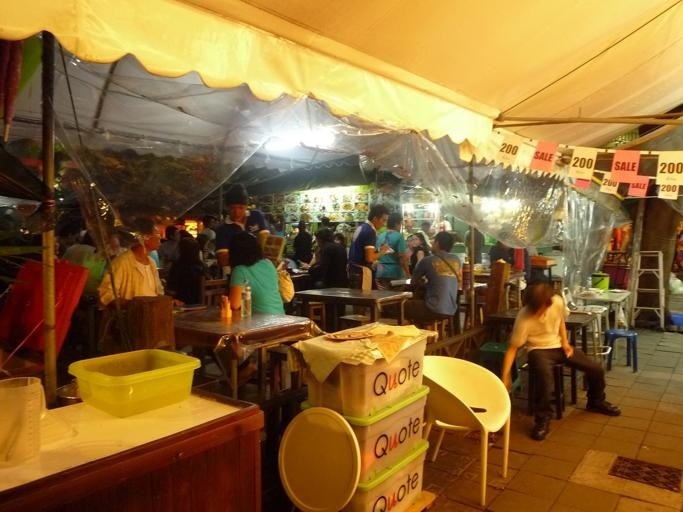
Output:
[
  {"xmin": 531, "ymin": 418, "xmax": 551, "ymax": 440},
  {"xmin": 586, "ymin": 401, "xmax": 621, "ymax": 417}
]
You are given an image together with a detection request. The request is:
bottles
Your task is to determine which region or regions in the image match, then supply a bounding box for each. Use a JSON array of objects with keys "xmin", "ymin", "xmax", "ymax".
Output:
[{"xmin": 239, "ymin": 286, "xmax": 251, "ymax": 319}]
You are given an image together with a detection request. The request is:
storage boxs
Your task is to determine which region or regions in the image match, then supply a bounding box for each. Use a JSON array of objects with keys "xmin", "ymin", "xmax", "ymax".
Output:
[{"xmin": 66, "ymin": 347, "xmax": 202, "ymax": 419}]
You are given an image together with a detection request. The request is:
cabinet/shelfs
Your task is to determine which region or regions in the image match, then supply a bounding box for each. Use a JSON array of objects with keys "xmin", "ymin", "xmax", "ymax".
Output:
[{"xmin": 0, "ymin": 387, "xmax": 266, "ymax": 512}]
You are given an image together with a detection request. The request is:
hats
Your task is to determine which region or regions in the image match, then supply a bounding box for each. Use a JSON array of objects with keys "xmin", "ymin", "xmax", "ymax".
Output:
[{"xmin": 224, "ymin": 183, "xmax": 249, "ymax": 206}]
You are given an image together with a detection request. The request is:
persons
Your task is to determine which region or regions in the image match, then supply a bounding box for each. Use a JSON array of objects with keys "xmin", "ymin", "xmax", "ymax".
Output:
[
  {"xmin": 216, "ymin": 184, "xmax": 266, "ymax": 281},
  {"xmin": 464, "ymin": 225, "xmax": 483, "ymax": 263},
  {"xmin": 214, "ymin": 231, "xmax": 281, "ymax": 388},
  {"xmin": 65, "ymin": 213, "xmax": 286, "ymax": 305},
  {"xmin": 403, "ymin": 232, "xmax": 460, "ymax": 321},
  {"xmin": 502, "ymin": 278, "xmax": 620, "ymax": 440},
  {"xmin": 488, "ymin": 223, "xmax": 531, "ymax": 302},
  {"xmin": 292, "ymin": 205, "xmax": 451, "ymax": 288}
]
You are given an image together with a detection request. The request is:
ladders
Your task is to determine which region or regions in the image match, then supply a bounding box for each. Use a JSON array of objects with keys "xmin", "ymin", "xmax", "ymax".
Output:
[{"xmin": 628, "ymin": 250, "xmax": 664, "ymax": 331}]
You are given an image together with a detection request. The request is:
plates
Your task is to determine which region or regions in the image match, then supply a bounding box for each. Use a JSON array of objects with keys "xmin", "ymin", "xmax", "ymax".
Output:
[
  {"xmin": 579, "ymin": 287, "xmax": 602, "ymax": 296},
  {"xmin": 610, "ymin": 288, "xmax": 627, "ymax": 294}
]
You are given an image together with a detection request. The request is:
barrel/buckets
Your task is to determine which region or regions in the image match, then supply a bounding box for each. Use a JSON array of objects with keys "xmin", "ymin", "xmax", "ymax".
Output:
[{"xmin": 57, "ymin": 383, "xmax": 83, "ymax": 406}]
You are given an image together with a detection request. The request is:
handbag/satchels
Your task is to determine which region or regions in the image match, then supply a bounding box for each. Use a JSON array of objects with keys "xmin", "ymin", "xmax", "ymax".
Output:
[{"xmin": 276, "ymin": 270, "xmax": 295, "ymax": 304}]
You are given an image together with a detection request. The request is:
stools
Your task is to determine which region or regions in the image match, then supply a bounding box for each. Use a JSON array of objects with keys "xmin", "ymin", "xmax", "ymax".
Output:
[{"xmin": 151, "ymin": 259, "xmax": 640, "ymax": 511}]
